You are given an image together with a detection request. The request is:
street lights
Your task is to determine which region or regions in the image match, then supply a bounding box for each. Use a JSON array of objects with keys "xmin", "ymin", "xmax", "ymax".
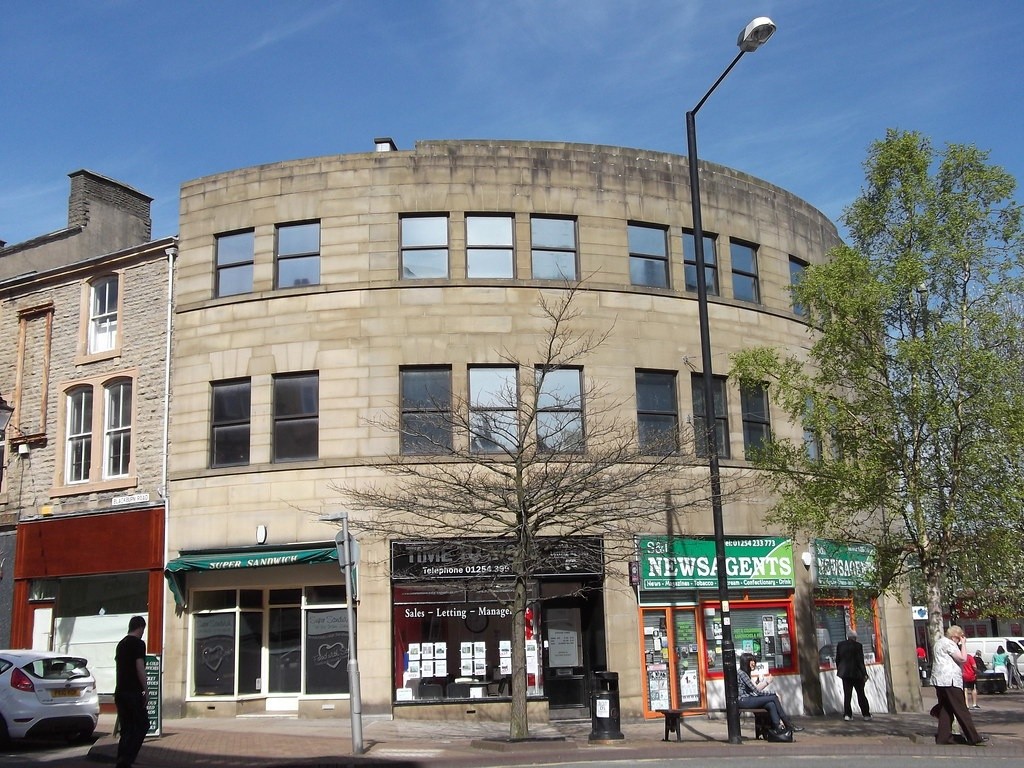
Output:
[
  {"xmin": 322, "ymin": 510, "xmax": 363, "ymax": 755},
  {"xmin": 684, "ymin": 17, "xmax": 778, "ymax": 746}
]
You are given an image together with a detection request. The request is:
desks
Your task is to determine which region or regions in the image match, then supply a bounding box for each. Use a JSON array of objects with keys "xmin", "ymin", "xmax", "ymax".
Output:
[{"xmin": 457, "ymin": 681, "xmax": 492, "ymax": 699}]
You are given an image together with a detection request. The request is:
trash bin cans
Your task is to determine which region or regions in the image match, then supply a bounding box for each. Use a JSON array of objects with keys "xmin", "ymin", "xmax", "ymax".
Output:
[
  {"xmin": 920, "ymin": 662, "xmax": 932, "ymax": 687},
  {"xmin": 588, "ymin": 670, "xmax": 625, "ymax": 741}
]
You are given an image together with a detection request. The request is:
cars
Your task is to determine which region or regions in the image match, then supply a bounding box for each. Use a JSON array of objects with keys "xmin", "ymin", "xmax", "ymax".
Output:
[
  {"xmin": 0, "ymin": 649, "xmax": 101, "ymax": 748},
  {"xmin": 277, "ymin": 645, "xmax": 301, "ymax": 685}
]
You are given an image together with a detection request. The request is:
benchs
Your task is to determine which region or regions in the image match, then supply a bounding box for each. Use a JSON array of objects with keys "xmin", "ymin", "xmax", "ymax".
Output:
[{"xmin": 655, "ymin": 708, "xmax": 777, "ymax": 743}]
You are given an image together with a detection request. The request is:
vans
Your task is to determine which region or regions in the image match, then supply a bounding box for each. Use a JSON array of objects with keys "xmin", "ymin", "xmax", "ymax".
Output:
[{"xmin": 962, "ymin": 636, "xmax": 1024, "ymax": 676}]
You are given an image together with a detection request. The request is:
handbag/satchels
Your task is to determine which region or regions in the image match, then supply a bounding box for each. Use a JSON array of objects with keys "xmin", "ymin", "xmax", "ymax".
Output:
[{"xmin": 768, "ymin": 729, "xmax": 793, "ymax": 742}]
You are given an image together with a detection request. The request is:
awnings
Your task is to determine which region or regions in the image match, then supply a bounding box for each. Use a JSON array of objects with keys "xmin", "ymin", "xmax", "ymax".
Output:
[{"xmin": 164, "ymin": 546, "xmax": 357, "ymax": 607}]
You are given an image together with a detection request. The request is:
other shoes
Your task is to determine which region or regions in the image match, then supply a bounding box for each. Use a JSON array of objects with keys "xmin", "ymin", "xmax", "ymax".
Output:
[
  {"xmin": 844, "ymin": 715, "xmax": 854, "ymax": 721},
  {"xmin": 979, "ymin": 738, "xmax": 989, "ymax": 741},
  {"xmin": 972, "ymin": 705, "xmax": 980, "ymax": 709},
  {"xmin": 864, "ymin": 715, "xmax": 873, "ymax": 721},
  {"xmin": 786, "ymin": 720, "xmax": 804, "ymax": 732}
]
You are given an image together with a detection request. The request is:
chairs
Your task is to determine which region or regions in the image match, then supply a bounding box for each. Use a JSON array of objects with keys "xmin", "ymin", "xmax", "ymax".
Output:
[{"xmin": 418, "ymin": 674, "xmax": 507, "ymax": 697}]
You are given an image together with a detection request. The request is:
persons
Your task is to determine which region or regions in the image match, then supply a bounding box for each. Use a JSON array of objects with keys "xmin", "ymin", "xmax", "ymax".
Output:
[
  {"xmin": 114, "ymin": 616, "xmax": 150, "ymax": 768},
  {"xmin": 917, "ymin": 638, "xmax": 927, "ymax": 662},
  {"xmin": 836, "ymin": 631, "xmax": 873, "ymax": 720},
  {"xmin": 959, "ymin": 644, "xmax": 981, "ymax": 709},
  {"xmin": 992, "ymin": 646, "xmax": 1010, "ymax": 689},
  {"xmin": 930, "ymin": 626, "xmax": 989, "ymax": 745},
  {"xmin": 973, "ymin": 650, "xmax": 988, "ymax": 672},
  {"xmin": 737, "ymin": 653, "xmax": 804, "ymax": 732}
]
